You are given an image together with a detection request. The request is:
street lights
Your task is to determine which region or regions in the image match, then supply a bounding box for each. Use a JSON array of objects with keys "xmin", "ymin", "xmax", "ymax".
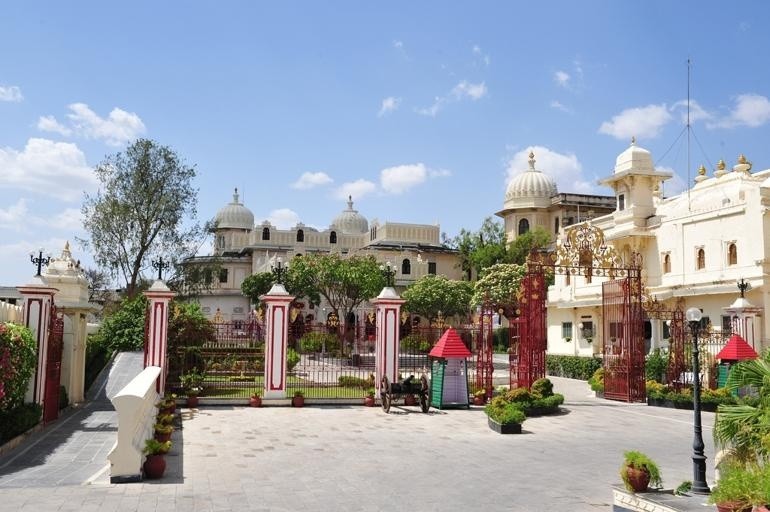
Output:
[
  {"xmin": 269, "ymin": 256, "xmax": 290, "ymax": 284},
  {"xmin": 685, "ymin": 307, "xmax": 714, "ymax": 494},
  {"xmin": 351, "ymin": 307, "xmax": 361, "ymax": 366},
  {"xmin": 378, "ymin": 260, "xmax": 399, "ymax": 286},
  {"xmin": 149, "ymin": 250, "xmax": 170, "ymax": 280},
  {"xmin": 320, "ymin": 306, "xmax": 328, "ymax": 354},
  {"xmin": 27, "ymin": 246, "xmax": 52, "ymax": 277},
  {"xmin": 734, "ymin": 273, "xmax": 751, "ymax": 297}
]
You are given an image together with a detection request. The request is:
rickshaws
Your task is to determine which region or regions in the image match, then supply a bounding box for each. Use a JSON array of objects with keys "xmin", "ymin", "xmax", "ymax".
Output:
[{"xmin": 377, "ymin": 374, "xmax": 433, "ymax": 415}]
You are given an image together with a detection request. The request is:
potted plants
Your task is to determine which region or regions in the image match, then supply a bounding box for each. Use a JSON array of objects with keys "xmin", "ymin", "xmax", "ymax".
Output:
[
  {"xmin": 293, "ymin": 391, "xmax": 305, "ymax": 407},
  {"xmin": 141, "ymin": 388, "xmax": 199, "ymax": 480},
  {"xmin": 249, "ymin": 389, "xmax": 261, "ymax": 406},
  {"xmin": 621, "ymin": 450, "xmax": 663, "ymax": 495},
  {"xmin": 365, "ymin": 388, "xmax": 376, "ymax": 406},
  {"xmin": 470, "ymin": 377, "xmax": 564, "ymax": 434},
  {"xmin": 707, "ymin": 460, "xmax": 770, "ymax": 512}
]
